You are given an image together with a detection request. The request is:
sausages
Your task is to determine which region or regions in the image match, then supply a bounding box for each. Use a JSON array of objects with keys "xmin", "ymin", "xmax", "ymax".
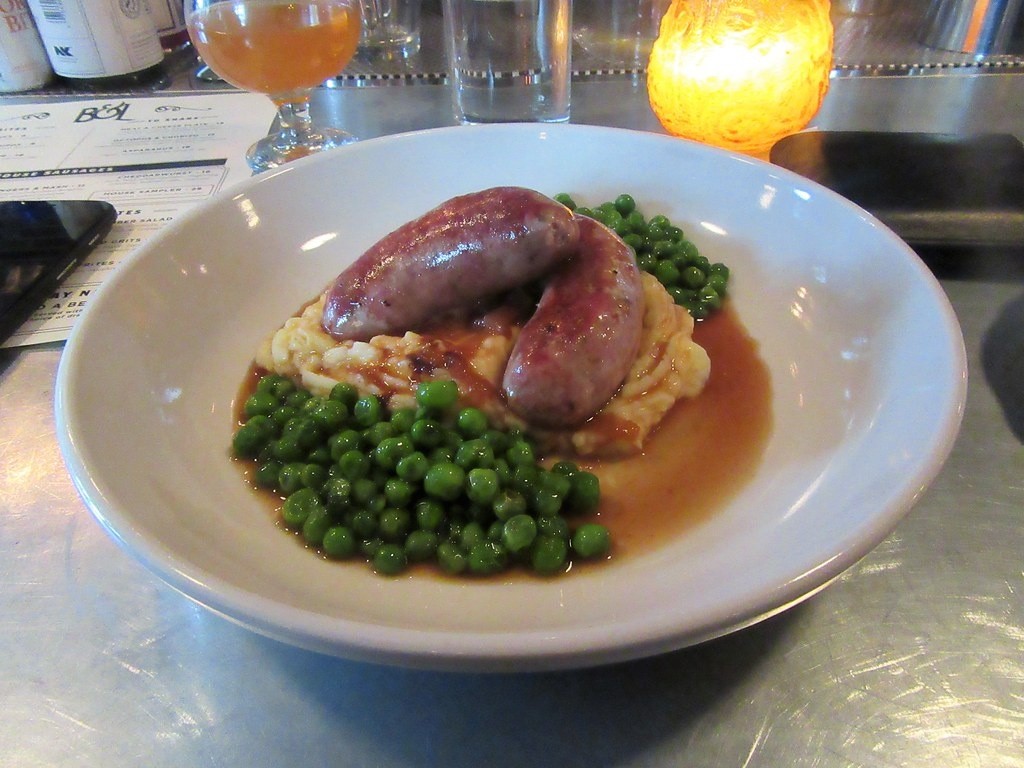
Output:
[
  {"xmin": 503, "ymin": 210, "xmax": 644, "ymax": 431},
  {"xmin": 321, "ymin": 185, "xmax": 579, "ymax": 343}
]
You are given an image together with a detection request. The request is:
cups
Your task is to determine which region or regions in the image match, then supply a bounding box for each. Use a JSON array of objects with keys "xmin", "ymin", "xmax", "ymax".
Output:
[
  {"xmin": 443, "ymin": 0, "xmax": 574, "ymax": 126},
  {"xmin": 346, "ymin": 0, "xmax": 422, "ymax": 63},
  {"xmin": 573, "ymin": 0, "xmax": 672, "ymax": 64}
]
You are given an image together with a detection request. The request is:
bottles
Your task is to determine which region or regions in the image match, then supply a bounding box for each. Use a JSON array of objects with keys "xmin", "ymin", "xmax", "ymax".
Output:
[
  {"xmin": 27, "ymin": 0, "xmax": 172, "ymax": 96},
  {"xmin": 0, "ymin": 0, "xmax": 56, "ymax": 95},
  {"xmin": 142, "ymin": 0, "xmax": 192, "ymax": 53}
]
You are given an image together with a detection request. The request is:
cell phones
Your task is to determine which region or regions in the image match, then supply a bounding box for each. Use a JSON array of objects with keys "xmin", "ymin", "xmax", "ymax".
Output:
[{"xmin": 0, "ymin": 200, "xmax": 117, "ymax": 347}]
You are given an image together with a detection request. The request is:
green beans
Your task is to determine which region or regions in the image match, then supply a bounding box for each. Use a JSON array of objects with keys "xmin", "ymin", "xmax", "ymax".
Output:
[
  {"xmin": 550, "ymin": 192, "xmax": 730, "ymax": 319},
  {"xmin": 234, "ymin": 372, "xmax": 608, "ymax": 574}
]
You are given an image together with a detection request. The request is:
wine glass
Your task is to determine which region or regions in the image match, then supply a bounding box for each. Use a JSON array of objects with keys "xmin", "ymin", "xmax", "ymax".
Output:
[{"xmin": 181, "ymin": 0, "xmax": 363, "ymax": 174}]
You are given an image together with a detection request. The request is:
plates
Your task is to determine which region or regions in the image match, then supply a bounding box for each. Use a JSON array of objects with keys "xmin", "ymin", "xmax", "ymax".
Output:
[{"xmin": 48, "ymin": 123, "xmax": 969, "ymax": 674}]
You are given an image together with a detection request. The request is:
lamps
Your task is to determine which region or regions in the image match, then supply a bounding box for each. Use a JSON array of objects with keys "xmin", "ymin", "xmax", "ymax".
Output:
[{"xmin": 645, "ymin": 1, "xmax": 834, "ymax": 162}]
